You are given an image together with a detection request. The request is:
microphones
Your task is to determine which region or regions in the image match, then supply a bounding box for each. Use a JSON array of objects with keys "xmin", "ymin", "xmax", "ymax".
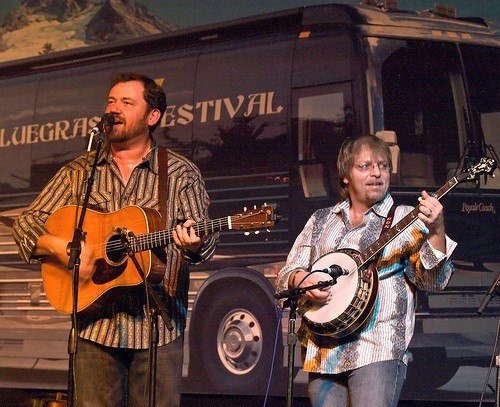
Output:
[
  {"xmin": 92, "ymin": 113, "xmax": 114, "ymax": 134},
  {"xmin": 322, "ymin": 264, "xmax": 349, "ymax": 276},
  {"xmin": 114, "ymin": 226, "xmax": 134, "ymax": 237}
]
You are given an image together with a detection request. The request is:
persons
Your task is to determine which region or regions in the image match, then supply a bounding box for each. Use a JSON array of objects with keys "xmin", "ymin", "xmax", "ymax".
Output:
[
  {"xmin": 274, "ymin": 131, "xmax": 458, "ymax": 407},
  {"xmin": 11, "ymin": 71, "xmax": 220, "ymax": 407}
]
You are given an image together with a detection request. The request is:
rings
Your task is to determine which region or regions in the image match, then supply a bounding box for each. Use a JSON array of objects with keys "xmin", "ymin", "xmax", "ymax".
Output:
[{"xmin": 427, "ymin": 209, "xmax": 432, "ymax": 218}]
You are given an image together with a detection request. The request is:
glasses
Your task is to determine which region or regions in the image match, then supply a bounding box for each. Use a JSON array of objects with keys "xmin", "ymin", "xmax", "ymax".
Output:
[{"xmin": 355, "ymin": 162, "xmax": 392, "ymax": 171}]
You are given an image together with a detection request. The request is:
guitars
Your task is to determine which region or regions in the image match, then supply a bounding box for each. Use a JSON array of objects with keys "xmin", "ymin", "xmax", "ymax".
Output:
[
  {"xmin": 296, "ymin": 154, "xmax": 498, "ymax": 337},
  {"xmin": 40, "ymin": 202, "xmax": 282, "ymax": 320}
]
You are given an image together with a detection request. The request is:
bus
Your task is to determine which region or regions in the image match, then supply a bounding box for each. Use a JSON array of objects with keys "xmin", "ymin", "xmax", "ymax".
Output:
[{"xmin": 0, "ymin": 0, "xmax": 499, "ymax": 394}]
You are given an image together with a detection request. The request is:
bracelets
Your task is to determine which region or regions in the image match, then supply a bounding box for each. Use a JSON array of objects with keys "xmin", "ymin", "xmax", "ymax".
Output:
[{"xmin": 292, "ymin": 269, "xmax": 304, "ymax": 288}]
